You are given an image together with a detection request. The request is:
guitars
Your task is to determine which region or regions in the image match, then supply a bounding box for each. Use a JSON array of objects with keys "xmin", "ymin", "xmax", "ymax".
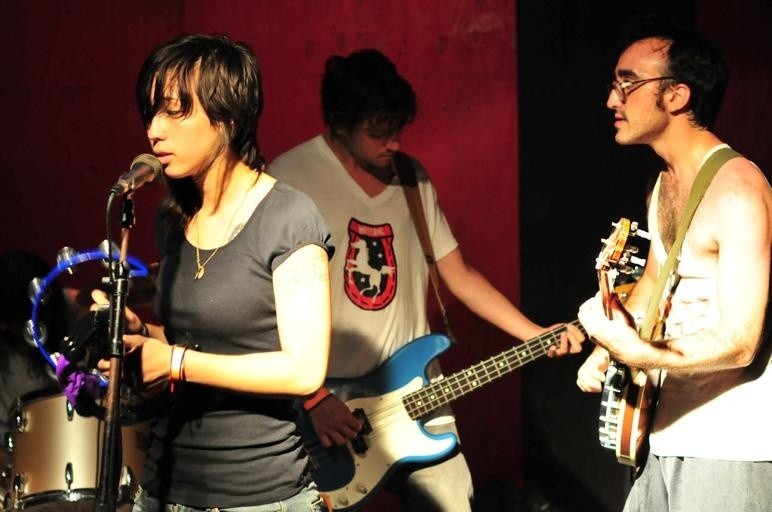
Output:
[
  {"xmin": 298, "ymin": 318, "xmax": 605, "ymax": 511},
  {"xmin": 595, "ymin": 218, "xmax": 679, "ymax": 468}
]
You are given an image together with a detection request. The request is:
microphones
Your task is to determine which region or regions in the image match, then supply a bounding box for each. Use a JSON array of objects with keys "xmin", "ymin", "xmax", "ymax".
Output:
[{"xmin": 110, "ymin": 153, "xmax": 162, "ymax": 195}]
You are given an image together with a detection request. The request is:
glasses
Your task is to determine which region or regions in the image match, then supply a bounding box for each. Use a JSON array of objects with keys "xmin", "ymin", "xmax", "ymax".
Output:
[{"xmin": 611, "ymin": 77, "xmax": 670, "ymax": 103}]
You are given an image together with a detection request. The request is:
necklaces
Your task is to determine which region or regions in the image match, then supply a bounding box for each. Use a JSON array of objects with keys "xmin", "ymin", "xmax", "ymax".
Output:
[{"xmin": 194, "ymin": 177, "xmax": 254, "ymax": 280}]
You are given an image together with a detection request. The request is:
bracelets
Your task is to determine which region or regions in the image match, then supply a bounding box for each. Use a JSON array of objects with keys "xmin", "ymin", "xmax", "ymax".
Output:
[
  {"xmin": 170, "ymin": 344, "xmax": 187, "ymax": 382},
  {"xmin": 301, "ymin": 388, "xmax": 328, "ymax": 409}
]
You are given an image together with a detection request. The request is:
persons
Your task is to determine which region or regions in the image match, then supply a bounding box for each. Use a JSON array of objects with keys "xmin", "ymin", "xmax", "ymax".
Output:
[
  {"xmin": 86, "ymin": 32, "xmax": 337, "ymax": 512},
  {"xmin": 575, "ymin": 33, "xmax": 771, "ymax": 511},
  {"xmin": 262, "ymin": 47, "xmax": 587, "ymax": 512}
]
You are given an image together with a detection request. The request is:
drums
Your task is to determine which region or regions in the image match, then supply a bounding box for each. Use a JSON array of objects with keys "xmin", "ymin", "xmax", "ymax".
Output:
[{"xmin": 0, "ymin": 384, "xmax": 154, "ymax": 512}]
